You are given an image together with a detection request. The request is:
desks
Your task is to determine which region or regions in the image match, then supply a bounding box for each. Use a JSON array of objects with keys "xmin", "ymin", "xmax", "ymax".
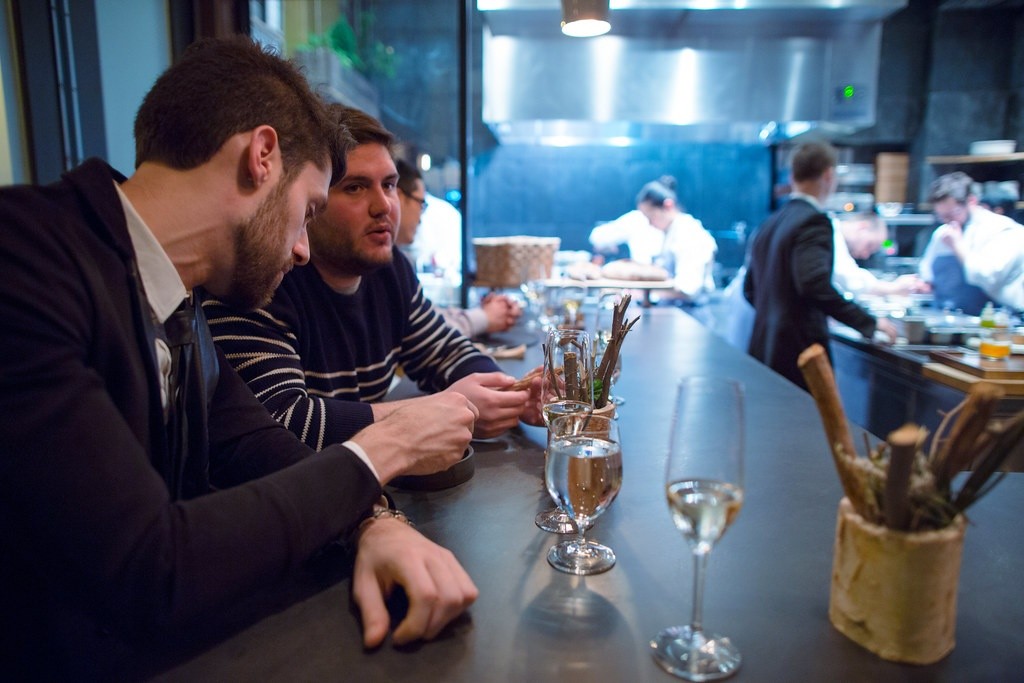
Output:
[{"xmin": 149, "ymin": 303, "xmax": 1024, "ymax": 683}]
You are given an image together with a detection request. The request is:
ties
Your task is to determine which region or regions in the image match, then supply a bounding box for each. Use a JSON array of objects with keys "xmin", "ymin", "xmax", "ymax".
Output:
[{"xmin": 164, "ymin": 300, "xmax": 209, "ymax": 493}]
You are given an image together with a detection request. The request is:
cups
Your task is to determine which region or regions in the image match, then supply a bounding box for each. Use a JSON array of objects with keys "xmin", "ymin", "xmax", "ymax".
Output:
[
  {"xmin": 982, "ymin": 324, "xmax": 1013, "ymax": 359},
  {"xmin": 905, "ymin": 319, "xmax": 926, "ymax": 344}
]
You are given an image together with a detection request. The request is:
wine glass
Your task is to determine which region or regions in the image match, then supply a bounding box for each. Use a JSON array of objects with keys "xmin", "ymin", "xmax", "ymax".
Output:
[
  {"xmin": 543, "ymin": 411, "xmax": 622, "ymax": 575},
  {"xmin": 529, "ymin": 329, "xmax": 599, "ymax": 533},
  {"xmin": 648, "ymin": 379, "xmax": 746, "ymax": 682}
]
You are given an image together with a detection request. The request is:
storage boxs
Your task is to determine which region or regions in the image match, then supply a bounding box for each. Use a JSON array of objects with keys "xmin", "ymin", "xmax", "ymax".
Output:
[{"xmin": 476, "ymin": 234, "xmax": 562, "ymax": 287}]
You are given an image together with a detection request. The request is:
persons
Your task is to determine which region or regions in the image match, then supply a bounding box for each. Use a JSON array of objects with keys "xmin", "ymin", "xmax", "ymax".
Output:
[
  {"xmin": 919, "ymin": 171, "xmax": 1024, "ymax": 328},
  {"xmin": 394, "ymin": 157, "xmax": 523, "ymax": 340},
  {"xmin": 724, "ymin": 212, "xmax": 931, "ymax": 353},
  {"xmin": 0, "ymin": 35, "xmax": 479, "ymax": 683},
  {"xmin": 589, "ymin": 175, "xmax": 718, "ymax": 330},
  {"xmin": 742, "ymin": 141, "xmax": 898, "ymax": 398},
  {"xmin": 201, "ymin": 104, "xmax": 566, "ymax": 452}
]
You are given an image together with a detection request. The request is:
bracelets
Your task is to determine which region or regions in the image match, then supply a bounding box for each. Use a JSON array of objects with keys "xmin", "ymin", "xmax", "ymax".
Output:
[{"xmin": 369, "ymin": 508, "xmax": 416, "ymax": 526}]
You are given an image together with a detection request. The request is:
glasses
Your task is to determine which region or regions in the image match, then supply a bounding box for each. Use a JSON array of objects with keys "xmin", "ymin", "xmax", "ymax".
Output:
[{"xmin": 402, "ymin": 192, "xmax": 428, "ymax": 215}]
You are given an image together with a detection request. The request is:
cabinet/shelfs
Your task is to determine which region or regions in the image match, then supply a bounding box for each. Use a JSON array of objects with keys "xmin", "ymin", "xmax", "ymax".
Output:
[
  {"xmin": 767, "ymin": 136, "xmax": 912, "ymax": 212},
  {"xmin": 926, "ymin": 151, "xmax": 1024, "ymax": 213}
]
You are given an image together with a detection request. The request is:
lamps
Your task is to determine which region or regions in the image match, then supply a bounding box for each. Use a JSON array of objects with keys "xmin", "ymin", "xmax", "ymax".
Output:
[{"xmin": 560, "ymin": 0, "xmax": 612, "ymax": 38}]
